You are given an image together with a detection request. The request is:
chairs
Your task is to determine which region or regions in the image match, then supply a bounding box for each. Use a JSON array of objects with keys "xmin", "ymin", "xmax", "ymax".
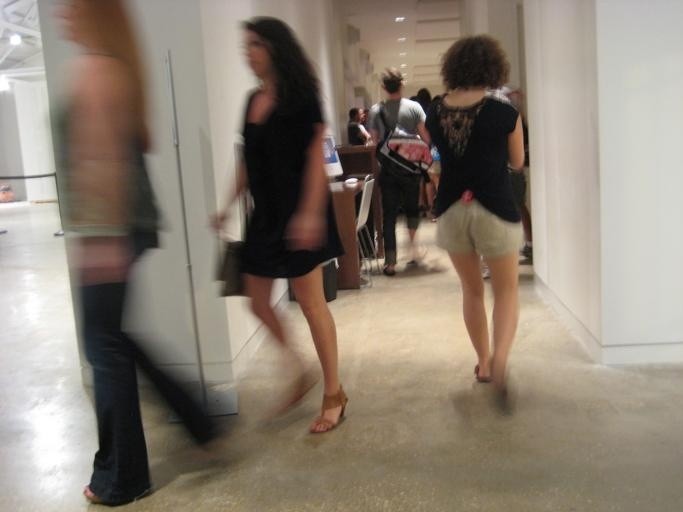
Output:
[{"xmin": 355, "ymin": 178, "xmax": 380, "ymax": 276}]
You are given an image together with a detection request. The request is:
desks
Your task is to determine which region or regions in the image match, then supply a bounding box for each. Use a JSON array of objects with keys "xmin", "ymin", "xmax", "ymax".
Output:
[
  {"xmin": 337, "ymin": 144, "xmax": 384, "ymax": 258},
  {"xmin": 328, "ymin": 180, "xmax": 363, "ymax": 288}
]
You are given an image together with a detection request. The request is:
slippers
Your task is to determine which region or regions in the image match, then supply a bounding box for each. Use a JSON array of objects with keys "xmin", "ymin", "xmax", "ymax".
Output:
[
  {"xmin": 383, "ymin": 262, "xmax": 396, "ymax": 276},
  {"xmin": 474, "ymin": 357, "xmax": 492, "ymax": 383}
]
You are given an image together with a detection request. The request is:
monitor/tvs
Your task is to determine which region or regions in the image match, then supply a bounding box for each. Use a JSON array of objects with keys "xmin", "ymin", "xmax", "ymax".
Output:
[{"xmin": 321, "ymin": 135, "xmax": 344, "ymax": 177}]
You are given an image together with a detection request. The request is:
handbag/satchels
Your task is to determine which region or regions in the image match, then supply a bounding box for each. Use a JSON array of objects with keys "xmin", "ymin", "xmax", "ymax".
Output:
[
  {"xmin": 211, "ymin": 238, "xmax": 249, "ymax": 299},
  {"xmin": 372, "ymin": 124, "xmax": 435, "ymax": 186}
]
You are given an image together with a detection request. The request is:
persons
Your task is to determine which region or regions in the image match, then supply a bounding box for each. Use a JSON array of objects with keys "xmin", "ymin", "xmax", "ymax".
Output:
[
  {"xmin": 45, "ymin": 0, "xmax": 249, "ymax": 506},
  {"xmin": 423, "ymin": 34, "xmax": 527, "ymax": 418},
  {"xmin": 209, "ymin": 14, "xmax": 348, "ymax": 434},
  {"xmin": 411, "ymin": 87, "xmax": 446, "ymax": 221},
  {"xmin": 368, "ymin": 70, "xmax": 431, "ymax": 275},
  {"xmin": 346, "ymin": 107, "xmax": 383, "ymax": 259},
  {"xmin": 510, "ymin": 91, "xmax": 532, "ymax": 264}
]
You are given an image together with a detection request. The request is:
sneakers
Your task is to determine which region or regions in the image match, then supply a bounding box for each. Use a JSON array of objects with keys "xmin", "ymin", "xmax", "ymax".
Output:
[{"xmin": 479, "ymin": 243, "xmax": 534, "ymax": 279}]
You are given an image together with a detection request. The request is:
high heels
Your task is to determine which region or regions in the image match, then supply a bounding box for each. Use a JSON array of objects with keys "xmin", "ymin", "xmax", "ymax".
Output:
[{"xmin": 308, "ymin": 384, "xmax": 348, "ymax": 433}]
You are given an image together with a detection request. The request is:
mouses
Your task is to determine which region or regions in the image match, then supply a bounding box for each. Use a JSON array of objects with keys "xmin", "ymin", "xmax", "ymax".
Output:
[{"xmin": 345, "ymin": 177, "xmax": 358, "ymax": 183}]
